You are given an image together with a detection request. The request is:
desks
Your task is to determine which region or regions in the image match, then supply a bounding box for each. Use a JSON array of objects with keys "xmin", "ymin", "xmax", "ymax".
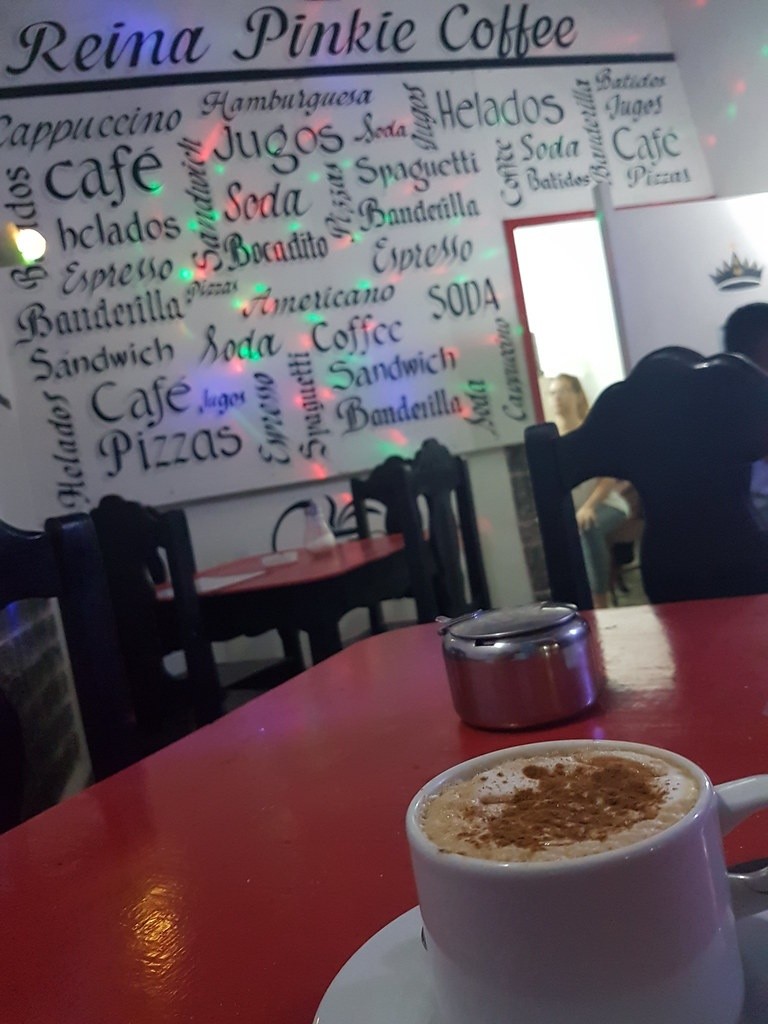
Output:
[
  {"xmin": 150, "ymin": 534, "xmax": 429, "ymax": 672},
  {"xmin": 0, "ymin": 593, "xmax": 768, "ymax": 1024}
]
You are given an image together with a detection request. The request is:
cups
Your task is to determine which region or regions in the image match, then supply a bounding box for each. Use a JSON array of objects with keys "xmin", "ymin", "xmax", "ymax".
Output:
[
  {"xmin": 436, "ymin": 600, "xmax": 595, "ymax": 732},
  {"xmin": 402, "ymin": 739, "xmax": 768, "ymax": 1024}
]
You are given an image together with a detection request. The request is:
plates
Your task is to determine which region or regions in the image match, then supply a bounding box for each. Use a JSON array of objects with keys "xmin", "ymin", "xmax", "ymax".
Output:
[{"xmin": 310, "ymin": 896, "xmax": 768, "ymax": 1024}]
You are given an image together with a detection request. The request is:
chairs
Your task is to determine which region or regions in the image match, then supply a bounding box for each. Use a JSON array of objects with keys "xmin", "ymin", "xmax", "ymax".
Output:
[{"xmin": 0, "ymin": 424, "xmax": 591, "ymax": 826}]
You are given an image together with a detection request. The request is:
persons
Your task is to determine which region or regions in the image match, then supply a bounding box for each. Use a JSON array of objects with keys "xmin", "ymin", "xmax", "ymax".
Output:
[
  {"xmin": 545, "ymin": 374, "xmax": 632, "ymax": 607},
  {"xmin": 725, "ymin": 302, "xmax": 768, "ymax": 532}
]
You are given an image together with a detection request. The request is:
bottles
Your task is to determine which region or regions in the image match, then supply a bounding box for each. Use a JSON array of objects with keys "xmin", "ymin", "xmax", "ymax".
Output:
[{"xmin": 305, "ymin": 500, "xmax": 336, "ymax": 555}]
